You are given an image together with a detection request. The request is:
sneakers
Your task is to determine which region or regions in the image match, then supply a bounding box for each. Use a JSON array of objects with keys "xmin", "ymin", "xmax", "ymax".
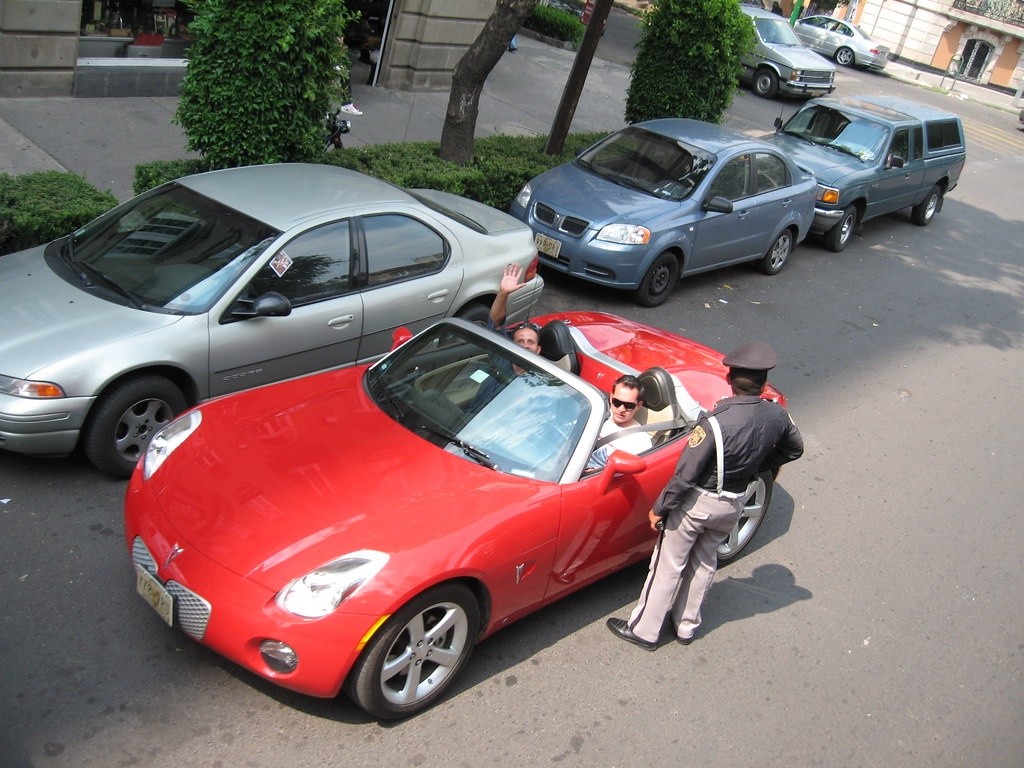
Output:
[{"xmin": 339, "ymin": 102, "xmax": 363, "ymax": 116}]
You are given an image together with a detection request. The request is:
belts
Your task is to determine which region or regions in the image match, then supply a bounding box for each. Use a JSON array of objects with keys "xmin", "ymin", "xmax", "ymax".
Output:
[{"xmin": 693, "ymin": 486, "xmax": 742, "ymax": 501}]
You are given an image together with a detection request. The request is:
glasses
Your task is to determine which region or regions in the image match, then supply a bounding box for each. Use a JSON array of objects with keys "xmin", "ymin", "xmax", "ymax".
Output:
[
  {"xmin": 516, "ymin": 323, "xmax": 542, "ymax": 333},
  {"xmin": 612, "ymin": 397, "xmax": 638, "ymax": 411}
]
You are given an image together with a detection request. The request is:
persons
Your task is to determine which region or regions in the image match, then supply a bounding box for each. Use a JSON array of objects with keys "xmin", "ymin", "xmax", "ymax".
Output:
[
  {"xmin": 509, "ymin": 37, "xmax": 517, "ymax": 51},
  {"xmin": 577, "ymin": 373, "xmax": 653, "ymax": 475},
  {"xmin": 605, "ymin": 341, "xmax": 804, "ymax": 651},
  {"xmin": 337, "ymin": 33, "xmax": 363, "ymax": 115},
  {"xmin": 771, "ymin": 1, "xmax": 782, "ymax": 16},
  {"xmin": 467, "ymin": 263, "xmax": 548, "ymax": 426}
]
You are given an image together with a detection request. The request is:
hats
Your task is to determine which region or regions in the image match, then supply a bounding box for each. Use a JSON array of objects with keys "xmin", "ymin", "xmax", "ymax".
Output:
[{"xmin": 722, "ymin": 341, "xmax": 778, "ymax": 371}]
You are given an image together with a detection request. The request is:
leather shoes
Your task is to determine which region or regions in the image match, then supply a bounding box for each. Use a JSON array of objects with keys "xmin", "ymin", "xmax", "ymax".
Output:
[
  {"xmin": 607, "ymin": 617, "xmax": 661, "ymax": 652},
  {"xmin": 668, "ymin": 610, "xmax": 694, "ymax": 646}
]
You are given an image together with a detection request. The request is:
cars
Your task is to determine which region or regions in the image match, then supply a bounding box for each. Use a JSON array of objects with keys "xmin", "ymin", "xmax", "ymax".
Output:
[
  {"xmin": 0, "ymin": 164, "xmax": 552, "ymax": 482},
  {"xmin": 733, "ymin": 3, "xmax": 837, "ymax": 98},
  {"xmin": 513, "ymin": 116, "xmax": 821, "ymax": 309},
  {"xmin": 785, "ymin": 14, "xmax": 891, "ymax": 71}
]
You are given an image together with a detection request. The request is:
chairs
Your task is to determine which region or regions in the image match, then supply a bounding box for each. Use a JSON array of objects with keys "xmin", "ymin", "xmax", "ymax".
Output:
[
  {"xmin": 167, "ymin": 217, "xmax": 259, "ymax": 269},
  {"xmin": 632, "ymin": 367, "xmax": 682, "ymax": 447},
  {"xmin": 538, "ymin": 320, "xmax": 578, "ymax": 375}
]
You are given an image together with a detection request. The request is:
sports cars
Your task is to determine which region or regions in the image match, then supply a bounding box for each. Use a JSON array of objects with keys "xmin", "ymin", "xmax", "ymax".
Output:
[{"xmin": 123, "ymin": 312, "xmax": 790, "ymax": 723}]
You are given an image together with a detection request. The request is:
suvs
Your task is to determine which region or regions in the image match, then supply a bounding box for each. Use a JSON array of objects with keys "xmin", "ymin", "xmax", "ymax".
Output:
[{"xmin": 742, "ymin": 95, "xmax": 969, "ymax": 253}]
